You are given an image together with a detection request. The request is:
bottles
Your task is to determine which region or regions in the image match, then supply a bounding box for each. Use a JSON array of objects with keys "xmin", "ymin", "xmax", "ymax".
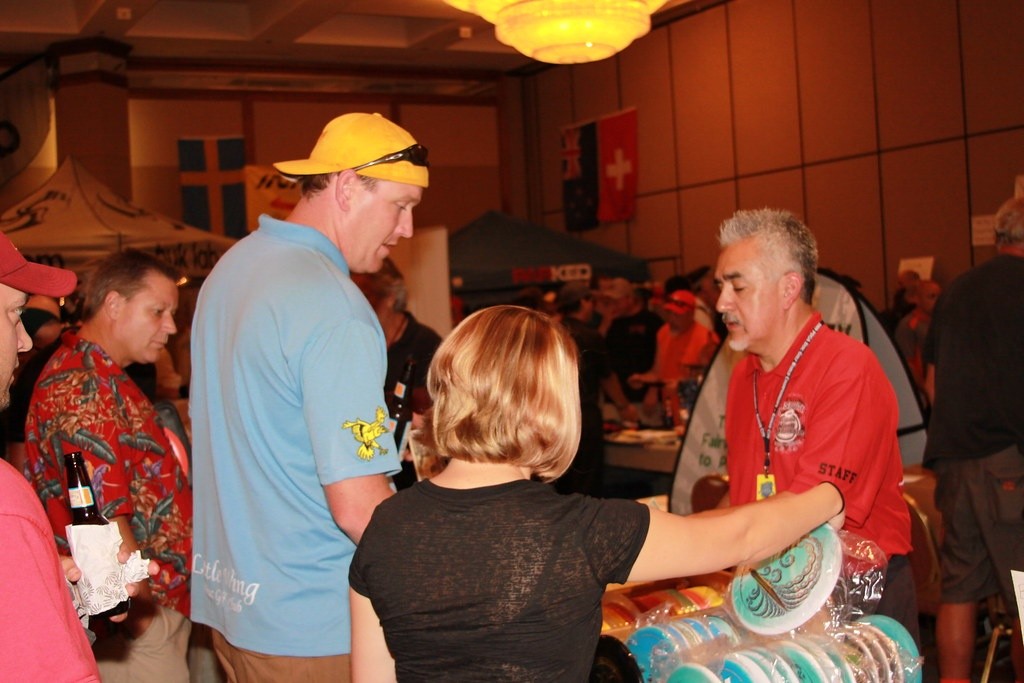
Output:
[
  {"xmin": 663, "ymin": 397, "xmax": 674, "ymax": 430},
  {"xmin": 62, "ymin": 450, "xmax": 132, "ymax": 619},
  {"xmin": 385, "ymin": 358, "xmax": 417, "ymax": 463}
]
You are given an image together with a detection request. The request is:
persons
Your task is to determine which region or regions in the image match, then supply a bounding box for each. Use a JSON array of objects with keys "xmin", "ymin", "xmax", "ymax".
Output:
[
  {"xmin": 922, "ymin": 200, "xmax": 1024, "ymax": 683},
  {"xmin": 347, "ymin": 306, "xmax": 845, "ymax": 683},
  {"xmin": 511, "ymin": 208, "xmax": 1012, "ymax": 668},
  {"xmin": 0, "ymin": 230, "xmax": 193, "ymax": 683},
  {"xmin": 191, "ymin": 111, "xmax": 429, "ymax": 683}
]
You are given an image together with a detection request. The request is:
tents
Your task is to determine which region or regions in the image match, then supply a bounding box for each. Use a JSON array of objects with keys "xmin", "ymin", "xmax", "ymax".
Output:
[
  {"xmin": 0, "ymin": 154, "xmax": 239, "ymax": 278},
  {"xmin": 450, "ymin": 207, "xmax": 650, "ymax": 297}
]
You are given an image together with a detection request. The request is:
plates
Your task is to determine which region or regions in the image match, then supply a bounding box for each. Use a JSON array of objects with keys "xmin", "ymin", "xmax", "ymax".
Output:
[{"xmin": 596, "ymin": 522, "xmax": 924, "ymax": 683}]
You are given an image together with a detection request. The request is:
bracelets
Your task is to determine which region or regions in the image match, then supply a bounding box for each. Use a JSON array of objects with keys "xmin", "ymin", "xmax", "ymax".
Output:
[{"xmin": 818, "ymin": 481, "xmax": 845, "ymax": 516}]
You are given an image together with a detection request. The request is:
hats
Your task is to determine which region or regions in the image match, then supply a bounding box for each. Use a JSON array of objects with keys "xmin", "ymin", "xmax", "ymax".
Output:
[
  {"xmin": 603, "ymin": 278, "xmax": 632, "ymax": 299},
  {"xmin": 0, "ymin": 230, "xmax": 78, "ymax": 298},
  {"xmin": 274, "ymin": 112, "xmax": 429, "ymax": 189},
  {"xmin": 663, "ymin": 289, "xmax": 695, "ymax": 314}
]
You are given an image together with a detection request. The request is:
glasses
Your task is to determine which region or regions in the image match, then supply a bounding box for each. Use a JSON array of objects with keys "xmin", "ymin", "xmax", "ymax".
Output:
[
  {"xmin": 664, "ymin": 295, "xmax": 692, "ymax": 308},
  {"xmin": 336, "ymin": 143, "xmax": 430, "ymax": 176}
]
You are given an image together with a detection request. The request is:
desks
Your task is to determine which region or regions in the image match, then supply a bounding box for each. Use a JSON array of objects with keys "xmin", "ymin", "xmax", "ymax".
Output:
[{"xmin": 599, "ymin": 426, "xmax": 679, "ymax": 474}]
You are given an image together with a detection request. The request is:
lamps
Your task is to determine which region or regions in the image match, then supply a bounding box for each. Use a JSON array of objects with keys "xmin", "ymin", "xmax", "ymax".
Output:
[{"xmin": 444, "ymin": 0, "xmax": 668, "ymax": 65}]
